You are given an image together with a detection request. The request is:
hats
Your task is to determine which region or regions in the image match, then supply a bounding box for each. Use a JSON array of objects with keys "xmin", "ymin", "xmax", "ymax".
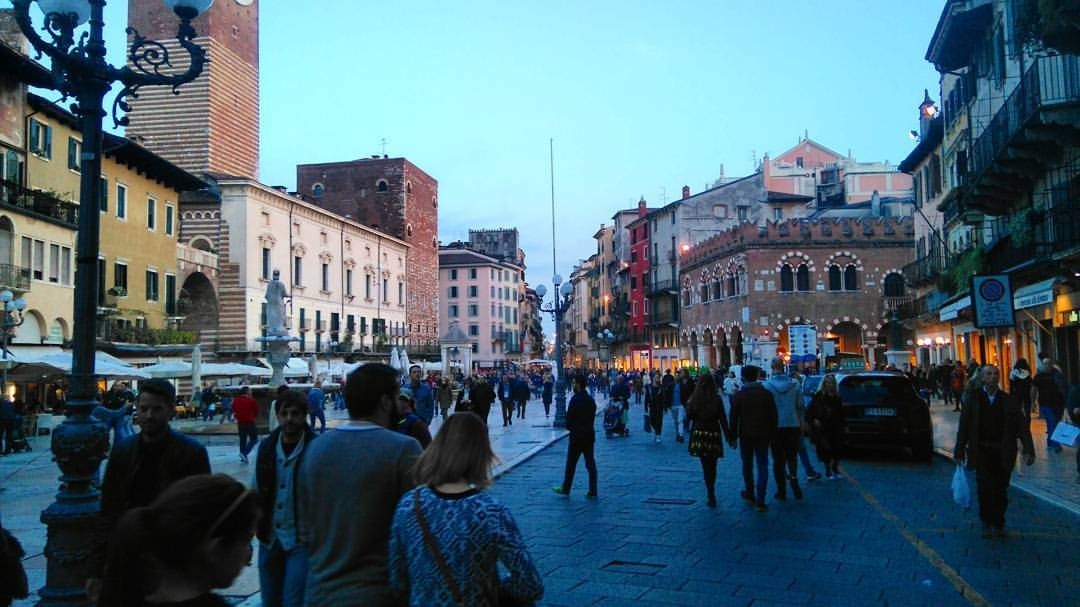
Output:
[{"xmin": 399, "ymin": 387, "xmax": 414, "ymax": 400}]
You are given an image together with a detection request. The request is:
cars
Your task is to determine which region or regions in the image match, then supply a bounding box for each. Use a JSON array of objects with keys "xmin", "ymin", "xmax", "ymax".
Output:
[{"xmin": 801, "ymin": 370, "xmax": 933, "ymax": 466}]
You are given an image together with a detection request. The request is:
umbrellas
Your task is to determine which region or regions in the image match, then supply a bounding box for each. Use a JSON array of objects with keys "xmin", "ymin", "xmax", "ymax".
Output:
[
  {"xmin": 87, "ymin": 346, "xmax": 271, "ymax": 400},
  {"xmin": 391, "ymin": 349, "xmax": 411, "ymax": 375}
]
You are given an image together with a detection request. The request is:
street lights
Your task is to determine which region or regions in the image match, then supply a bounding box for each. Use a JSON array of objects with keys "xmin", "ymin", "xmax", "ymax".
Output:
[
  {"xmin": 10, "ymin": 0, "xmax": 215, "ymax": 607},
  {"xmin": 0, "ymin": 290, "xmax": 27, "ymax": 384},
  {"xmin": 597, "ymin": 329, "xmax": 614, "ymax": 377},
  {"xmin": 534, "ymin": 273, "xmax": 574, "ymax": 428}
]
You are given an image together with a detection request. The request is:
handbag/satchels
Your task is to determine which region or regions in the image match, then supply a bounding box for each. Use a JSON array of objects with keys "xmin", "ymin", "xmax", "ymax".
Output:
[
  {"xmin": 1051, "ymin": 422, "xmax": 1080, "ymax": 448},
  {"xmin": 951, "ymin": 461, "xmax": 973, "ymax": 507},
  {"xmin": 643, "ymin": 415, "xmax": 652, "ymax": 433}
]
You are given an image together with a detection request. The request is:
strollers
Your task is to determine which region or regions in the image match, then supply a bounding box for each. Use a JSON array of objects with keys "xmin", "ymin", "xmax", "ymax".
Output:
[{"xmin": 604, "ymin": 394, "xmax": 629, "ymax": 439}]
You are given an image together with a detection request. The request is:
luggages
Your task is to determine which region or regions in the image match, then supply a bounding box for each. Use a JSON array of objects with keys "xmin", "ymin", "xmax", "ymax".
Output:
[{"xmin": 603, "ymin": 405, "xmax": 621, "ymax": 433}]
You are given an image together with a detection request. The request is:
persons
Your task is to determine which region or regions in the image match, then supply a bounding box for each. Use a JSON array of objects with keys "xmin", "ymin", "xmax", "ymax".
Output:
[
  {"xmin": 265, "ymin": 269, "xmax": 289, "ymax": 337},
  {"xmin": 0, "ymin": 353, "xmax": 1080, "ymax": 607}
]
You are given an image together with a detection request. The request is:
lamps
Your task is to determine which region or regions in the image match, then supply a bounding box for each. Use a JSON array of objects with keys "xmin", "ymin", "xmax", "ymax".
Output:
[{"xmin": 909, "ymin": 130, "xmax": 922, "ymax": 139}]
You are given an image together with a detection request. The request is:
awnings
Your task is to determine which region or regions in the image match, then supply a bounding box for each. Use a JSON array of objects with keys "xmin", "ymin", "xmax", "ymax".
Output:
[
  {"xmin": 940, "ymin": 296, "xmax": 973, "ymax": 323},
  {"xmin": 6, "ymin": 347, "xmax": 132, "ymax": 369},
  {"xmin": 256, "ymin": 357, "xmax": 464, "ymax": 371}
]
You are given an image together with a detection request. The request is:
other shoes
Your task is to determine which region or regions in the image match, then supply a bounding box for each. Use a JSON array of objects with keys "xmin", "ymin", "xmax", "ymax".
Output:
[
  {"xmin": 503, "ymin": 424, "xmax": 507, "ymax": 426},
  {"xmin": 774, "ymin": 492, "xmax": 786, "ymax": 500},
  {"xmin": 807, "ymin": 472, "xmax": 822, "ymax": 481},
  {"xmin": 679, "ymin": 436, "xmax": 684, "ymax": 443},
  {"xmin": 755, "ymin": 505, "xmax": 768, "ymax": 512},
  {"xmin": 833, "ymin": 474, "xmax": 843, "ymax": 480},
  {"xmin": 655, "ymin": 438, "xmax": 661, "ymax": 443},
  {"xmin": 706, "ymin": 497, "xmax": 717, "ymax": 507},
  {"xmin": 740, "ymin": 489, "xmax": 755, "ymax": 502},
  {"xmin": 676, "ymin": 435, "xmax": 679, "ymax": 441},
  {"xmin": 507, "ymin": 416, "xmax": 513, "ymax": 425},
  {"xmin": 825, "ymin": 473, "xmax": 834, "ymax": 480}
]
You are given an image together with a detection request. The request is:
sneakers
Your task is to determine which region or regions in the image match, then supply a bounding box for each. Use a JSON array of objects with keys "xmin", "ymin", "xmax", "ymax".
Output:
[
  {"xmin": 552, "ymin": 486, "xmax": 570, "ymax": 495},
  {"xmin": 239, "ymin": 452, "xmax": 248, "ymax": 463},
  {"xmin": 583, "ymin": 491, "xmax": 597, "ymax": 500}
]
